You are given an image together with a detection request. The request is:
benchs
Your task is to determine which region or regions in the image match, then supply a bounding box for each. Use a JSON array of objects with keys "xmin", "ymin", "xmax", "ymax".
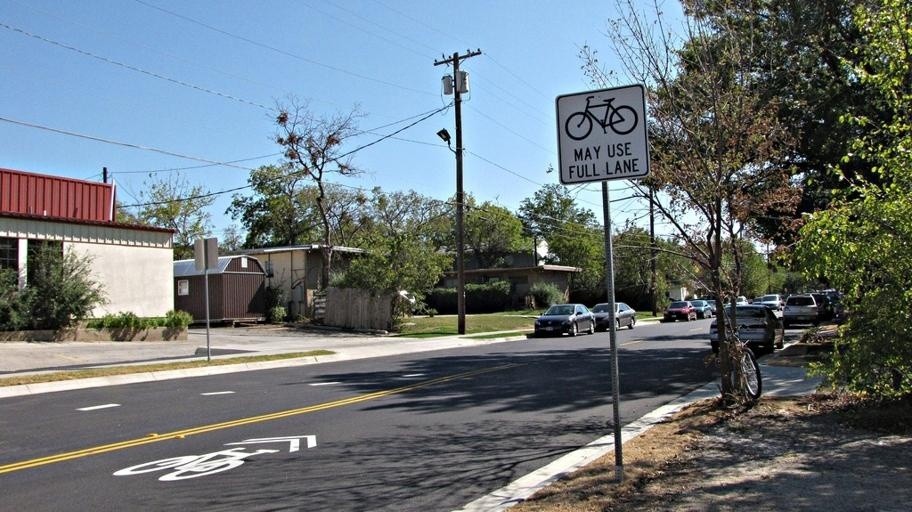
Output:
[{"xmin": 222, "ymin": 318, "xmax": 260, "ymax": 328}]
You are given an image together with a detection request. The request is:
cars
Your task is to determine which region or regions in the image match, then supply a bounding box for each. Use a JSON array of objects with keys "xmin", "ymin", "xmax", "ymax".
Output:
[
  {"xmin": 663, "ymin": 301, "xmax": 698, "ymax": 322},
  {"xmin": 589, "ymin": 301, "xmax": 638, "ymax": 331},
  {"xmin": 708, "ymin": 302, "xmax": 785, "ymax": 355},
  {"xmin": 688, "ymin": 289, "xmax": 842, "ymax": 319},
  {"xmin": 534, "ymin": 303, "xmax": 597, "ymax": 337}
]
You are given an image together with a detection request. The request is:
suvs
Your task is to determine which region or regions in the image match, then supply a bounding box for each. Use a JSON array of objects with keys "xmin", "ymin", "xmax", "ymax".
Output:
[{"xmin": 780, "ymin": 292, "xmax": 818, "ymax": 328}]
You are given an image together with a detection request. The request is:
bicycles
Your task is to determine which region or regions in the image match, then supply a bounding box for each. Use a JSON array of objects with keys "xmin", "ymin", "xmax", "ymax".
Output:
[{"xmin": 725, "ymin": 323, "xmax": 764, "ymax": 400}]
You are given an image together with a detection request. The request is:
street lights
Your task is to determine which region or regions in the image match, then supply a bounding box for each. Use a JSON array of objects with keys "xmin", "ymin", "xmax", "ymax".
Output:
[{"xmin": 437, "ymin": 128, "xmax": 469, "ymax": 334}]
